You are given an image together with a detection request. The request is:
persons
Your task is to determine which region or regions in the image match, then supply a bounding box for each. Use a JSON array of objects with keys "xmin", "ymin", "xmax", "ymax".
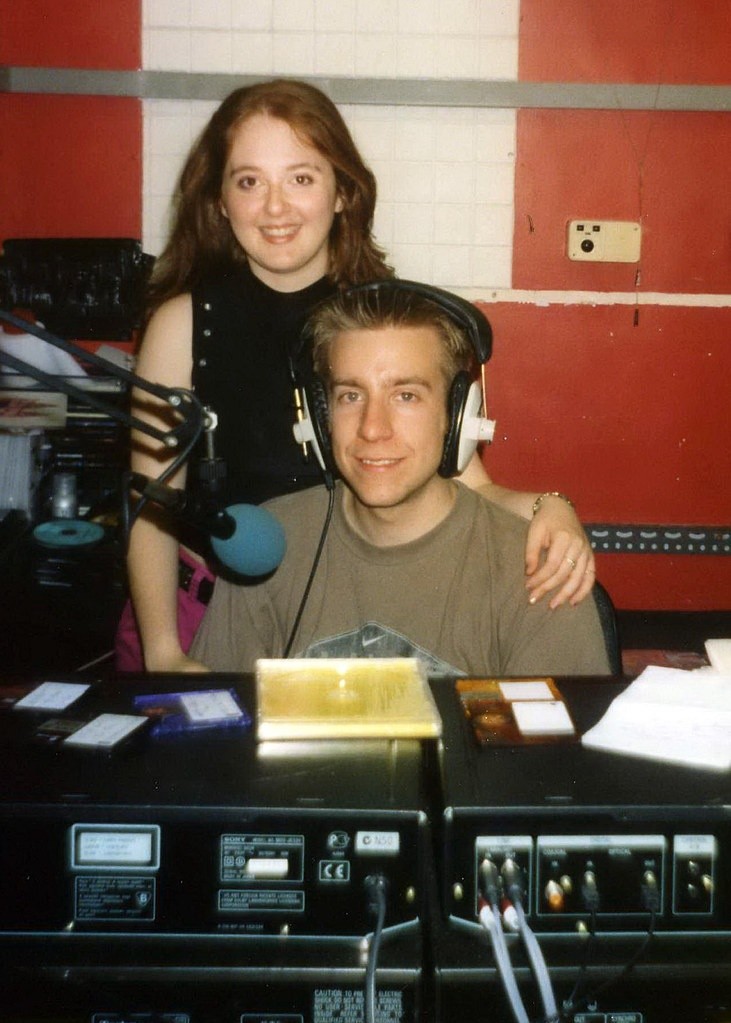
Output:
[
  {"xmin": 121, "ymin": 76, "xmax": 599, "ymax": 670},
  {"xmin": 187, "ymin": 288, "xmax": 615, "ymax": 677}
]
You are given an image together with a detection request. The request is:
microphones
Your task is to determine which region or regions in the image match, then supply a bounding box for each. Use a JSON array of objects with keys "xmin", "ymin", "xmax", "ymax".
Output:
[{"xmin": 124, "ymin": 470, "xmax": 286, "ymax": 576}]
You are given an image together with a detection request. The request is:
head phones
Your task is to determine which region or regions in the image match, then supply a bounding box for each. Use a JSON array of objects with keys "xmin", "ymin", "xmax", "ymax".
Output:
[{"xmin": 288, "ymin": 277, "xmax": 496, "ymax": 478}]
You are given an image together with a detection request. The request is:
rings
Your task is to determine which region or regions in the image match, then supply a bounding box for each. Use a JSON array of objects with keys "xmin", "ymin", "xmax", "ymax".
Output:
[
  {"xmin": 563, "ymin": 556, "xmax": 575, "ymax": 569},
  {"xmin": 584, "ymin": 569, "xmax": 597, "ymax": 574}
]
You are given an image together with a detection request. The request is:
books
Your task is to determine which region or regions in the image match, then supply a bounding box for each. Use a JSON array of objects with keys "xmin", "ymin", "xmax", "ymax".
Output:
[{"xmin": 253, "ymin": 652, "xmax": 441, "ymax": 740}]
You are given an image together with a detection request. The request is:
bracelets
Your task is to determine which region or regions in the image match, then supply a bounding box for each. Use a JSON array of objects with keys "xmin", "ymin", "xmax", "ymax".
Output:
[{"xmin": 532, "ymin": 491, "xmax": 578, "ymax": 518}]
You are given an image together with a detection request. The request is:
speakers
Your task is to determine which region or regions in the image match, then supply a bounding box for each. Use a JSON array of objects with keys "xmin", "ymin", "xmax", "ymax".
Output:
[{"xmin": 0, "ymin": 425, "xmax": 131, "ymax": 681}]
